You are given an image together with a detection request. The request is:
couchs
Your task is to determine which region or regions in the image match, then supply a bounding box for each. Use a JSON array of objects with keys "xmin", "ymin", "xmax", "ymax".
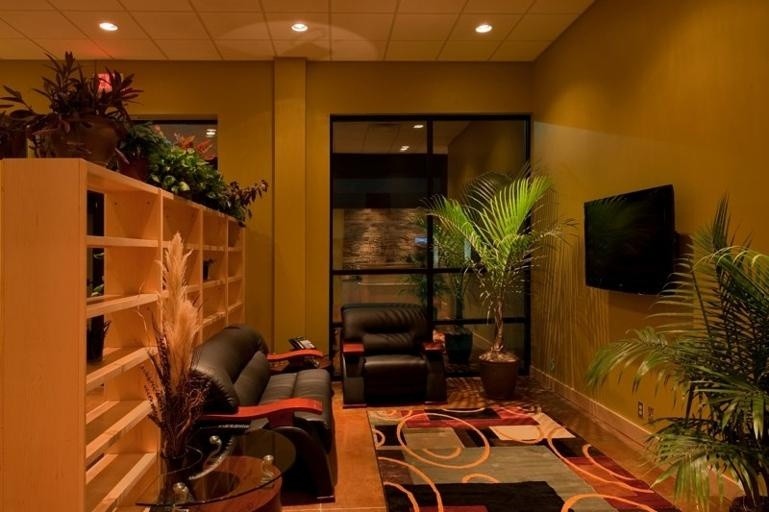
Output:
[{"xmin": 192, "ymin": 322, "xmax": 335, "ymax": 503}]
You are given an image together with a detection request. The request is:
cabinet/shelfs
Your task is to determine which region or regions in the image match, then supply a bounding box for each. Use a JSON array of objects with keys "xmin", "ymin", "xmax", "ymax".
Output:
[{"xmin": 0, "ymin": 157, "xmax": 247, "ymax": 511}]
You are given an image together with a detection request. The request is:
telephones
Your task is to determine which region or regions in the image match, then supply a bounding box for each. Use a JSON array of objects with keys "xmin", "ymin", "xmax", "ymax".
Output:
[{"xmin": 288, "ymin": 336, "xmax": 316, "ymax": 350}]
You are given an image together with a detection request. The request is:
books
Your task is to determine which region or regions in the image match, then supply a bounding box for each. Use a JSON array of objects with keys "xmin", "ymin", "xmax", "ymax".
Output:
[{"xmin": 289, "ymin": 336, "xmax": 315, "ymax": 349}]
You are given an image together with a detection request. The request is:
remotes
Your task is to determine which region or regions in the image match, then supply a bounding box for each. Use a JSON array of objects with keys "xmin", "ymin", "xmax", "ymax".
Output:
[{"xmin": 200, "ymin": 424, "xmax": 252, "ymax": 431}]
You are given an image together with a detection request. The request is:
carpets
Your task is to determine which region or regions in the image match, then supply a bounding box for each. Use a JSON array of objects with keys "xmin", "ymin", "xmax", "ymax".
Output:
[{"xmin": 366, "ymin": 405, "xmax": 682, "ymax": 512}]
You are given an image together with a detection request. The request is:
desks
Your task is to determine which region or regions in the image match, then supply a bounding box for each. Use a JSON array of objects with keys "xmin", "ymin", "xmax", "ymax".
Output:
[{"xmin": 138, "ymin": 425, "xmax": 297, "ymax": 512}]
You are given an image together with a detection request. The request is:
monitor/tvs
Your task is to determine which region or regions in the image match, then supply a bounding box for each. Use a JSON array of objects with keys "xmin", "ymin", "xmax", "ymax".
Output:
[{"xmin": 584, "ymin": 183, "xmax": 676, "ymax": 297}]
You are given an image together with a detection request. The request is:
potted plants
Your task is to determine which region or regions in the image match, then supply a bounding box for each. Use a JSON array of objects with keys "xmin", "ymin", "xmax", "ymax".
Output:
[
  {"xmin": 135, "ymin": 232, "xmax": 209, "ymax": 468},
  {"xmin": 0, "ymin": 51, "xmax": 144, "ymax": 170},
  {"xmin": 166, "ymin": 132, "xmax": 218, "ymax": 199},
  {"xmin": 420, "ymin": 158, "xmax": 579, "ymax": 399},
  {"xmin": 115, "ymin": 122, "xmax": 177, "ymax": 182},
  {"xmin": 402, "ymin": 240, "xmax": 453, "ymax": 322},
  {"xmin": 582, "ymin": 190, "xmax": 769, "ymax": 512},
  {"xmin": 396, "ymin": 212, "xmax": 474, "ymax": 364}
]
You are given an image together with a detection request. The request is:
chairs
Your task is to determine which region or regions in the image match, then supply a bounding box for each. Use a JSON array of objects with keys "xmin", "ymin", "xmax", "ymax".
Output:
[{"xmin": 341, "ymin": 303, "xmax": 446, "ymax": 405}]
